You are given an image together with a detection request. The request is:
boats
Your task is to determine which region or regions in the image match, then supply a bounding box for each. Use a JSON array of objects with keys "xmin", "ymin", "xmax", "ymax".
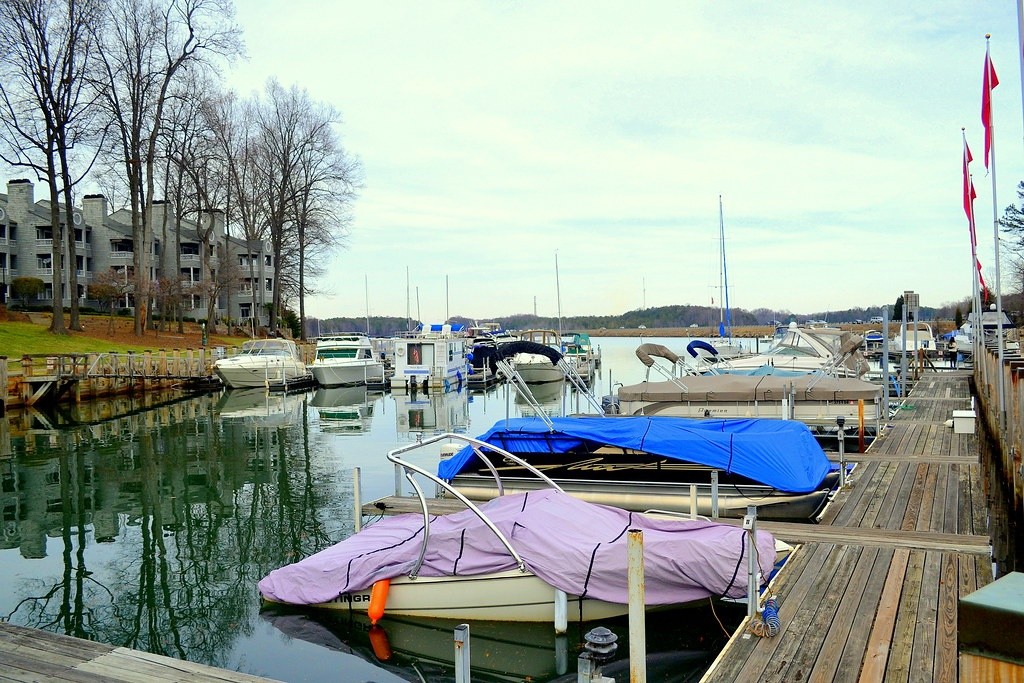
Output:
[
  {"xmin": 213, "ymin": 337, "xmax": 314, "ymax": 390},
  {"xmin": 560, "ymin": 331, "xmax": 597, "ymax": 383},
  {"xmin": 216, "ymin": 391, "xmax": 307, "ymax": 419},
  {"xmin": 436, "ymin": 340, "xmax": 856, "ymax": 526},
  {"xmin": 600, "ymin": 315, "xmax": 969, "ymax": 428},
  {"xmin": 305, "ymin": 335, "xmax": 385, "ymax": 389},
  {"xmin": 307, "ymin": 385, "xmax": 388, "ymax": 435},
  {"xmin": 512, "ymin": 328, "xmax": 567, "ymax": 383},
  {"xmin": 256, "ymin": 431, "xmax": 795, "ymax": 683},
  {"xmin": 398, "ymin": 322, "xmax": 516, "ymax": 391},
  {"xmin": 514, "ymin": 379, "xmax": 565, "ymax": 407}
]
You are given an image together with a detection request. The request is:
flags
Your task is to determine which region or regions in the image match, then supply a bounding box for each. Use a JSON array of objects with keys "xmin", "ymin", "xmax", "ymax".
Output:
[
  {"xmin": 961, "ymin": 139, "xmax": 987, "ymax": 300},
  {"xmin": 980, "ymin": 42, "xmax": 1000, "ymax": 172}
]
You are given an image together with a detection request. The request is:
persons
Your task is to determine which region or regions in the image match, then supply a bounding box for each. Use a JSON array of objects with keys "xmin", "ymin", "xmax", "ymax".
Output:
[{"xmin": 949, "ymin": 337, "xmax": 959, "ymax": 368}]
